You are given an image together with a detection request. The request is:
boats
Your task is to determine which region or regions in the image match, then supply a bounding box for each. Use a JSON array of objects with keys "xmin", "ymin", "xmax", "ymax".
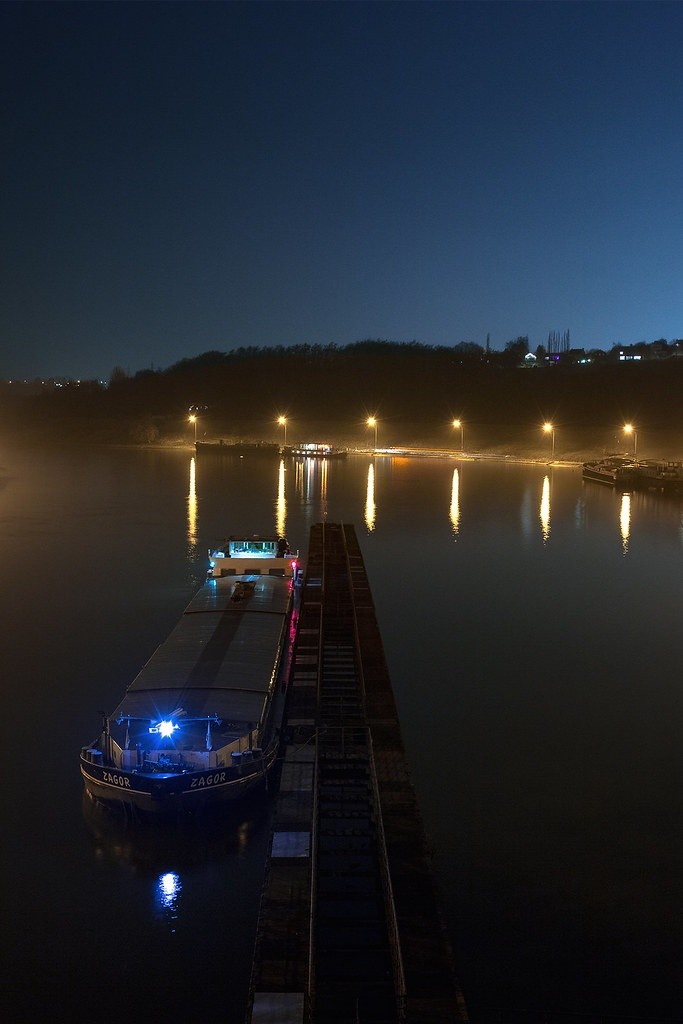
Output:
[
  {"xmin": 195, "ymin": 440, "xmax": 280, "ymax": 457},
  {"xmin": 282, "ymin": 440, "xmax": 350, "ymax": 460},
  {"xmin": 80, "ymin": 531, "xmax": 306, "ymax": 817},
  {"xmin": 582, "ymin": 455, "xmax": 683, "ymax": 498}
]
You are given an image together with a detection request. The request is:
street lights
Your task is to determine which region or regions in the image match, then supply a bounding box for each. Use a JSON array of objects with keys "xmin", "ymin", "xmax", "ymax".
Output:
[
  {"xmin": 188, "ymin": 415, "xmax": 197, "ymax": 442},
  {"xmin": 278, "ymin": 416, "xmax": 287, "ymax": 443},
  {"xmin": 622, "ymin": 424, "xmax": 637, "ymax": 454},
  {"xmin": 543, "ymin": 422, "xmax": 555, "ymax": 459},
  {"xmin": 366, "ymin": 416, "xmax": 378, "ymax": 448},
  {"xmin": 453, "ymin": 418, "xmax": 465, "ymax": 452}
]
